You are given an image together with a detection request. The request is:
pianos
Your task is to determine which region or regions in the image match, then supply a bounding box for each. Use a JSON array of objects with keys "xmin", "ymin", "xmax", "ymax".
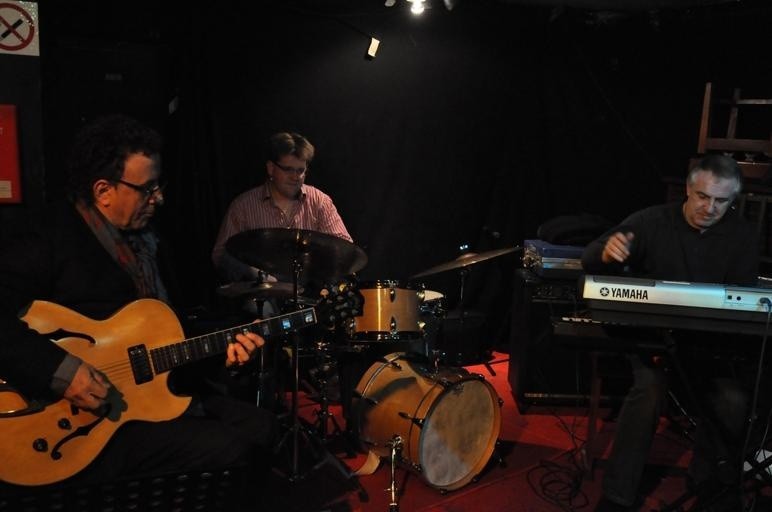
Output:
[{"xmin": 575, "ymin": 275, "xmax": 772, "ymax": 335}]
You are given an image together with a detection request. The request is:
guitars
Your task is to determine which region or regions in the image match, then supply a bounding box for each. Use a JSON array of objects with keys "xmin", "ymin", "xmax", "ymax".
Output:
[{"xmin": 0, "ymin": 278, "xmax": 364, "ymax": 486}]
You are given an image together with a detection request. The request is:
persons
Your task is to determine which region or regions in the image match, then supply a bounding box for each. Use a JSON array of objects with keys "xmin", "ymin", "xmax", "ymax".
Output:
[
  {"xmin": 0, "ymin": 116, "xmax": 267, "ymax": 511},
  {"xmin": 584, "ymin": 150, "xmax": 771, "ymax": 511},
  {"xmin": 203, "ymin": 130, "xmax": 356, "ymax": 334}
]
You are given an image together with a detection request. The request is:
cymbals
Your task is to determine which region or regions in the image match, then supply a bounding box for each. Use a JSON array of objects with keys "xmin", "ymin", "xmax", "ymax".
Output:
[
  {"xmin": 413, "ymin": 246, "xmax": 523, "ymax": 279},
  {"xmin": 217, "ymin": 283, "xmax": 305, "ymax": 300},
  {"xmin": 225, "ymin": 227, "xmax": 369, "ymax": 287}
]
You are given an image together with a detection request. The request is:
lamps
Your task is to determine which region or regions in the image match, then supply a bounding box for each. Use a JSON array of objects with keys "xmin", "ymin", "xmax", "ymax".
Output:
[
  {"xmin": 363, "ymin": 35, "xmax": 380, "ymax": 61},
  {"xmin": 407, "ymin": 0, "xmax": 426, "ymax": 15}
]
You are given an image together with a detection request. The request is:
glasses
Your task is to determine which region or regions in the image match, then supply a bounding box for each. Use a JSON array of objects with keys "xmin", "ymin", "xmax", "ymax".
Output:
[
  {"xmin": 118, "ymin": 180, "xmax": 163, "ymax": 194},
  {"xmin": 273, "ymin": 161, "xmax": 306, "ymax": 175}
]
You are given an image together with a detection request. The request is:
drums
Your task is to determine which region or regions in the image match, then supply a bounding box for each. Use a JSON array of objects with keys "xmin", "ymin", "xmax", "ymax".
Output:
[
  {"xmin": 350, "ymin": 279, "xmax": 421, "ymax": 342},
  {"xmin": 419, "ymin": 288, "xmax": 447, "ymax": 331},
  {"xmin": 350, "ymin": 350, "xmax": 503, "ymax": 494}
]
